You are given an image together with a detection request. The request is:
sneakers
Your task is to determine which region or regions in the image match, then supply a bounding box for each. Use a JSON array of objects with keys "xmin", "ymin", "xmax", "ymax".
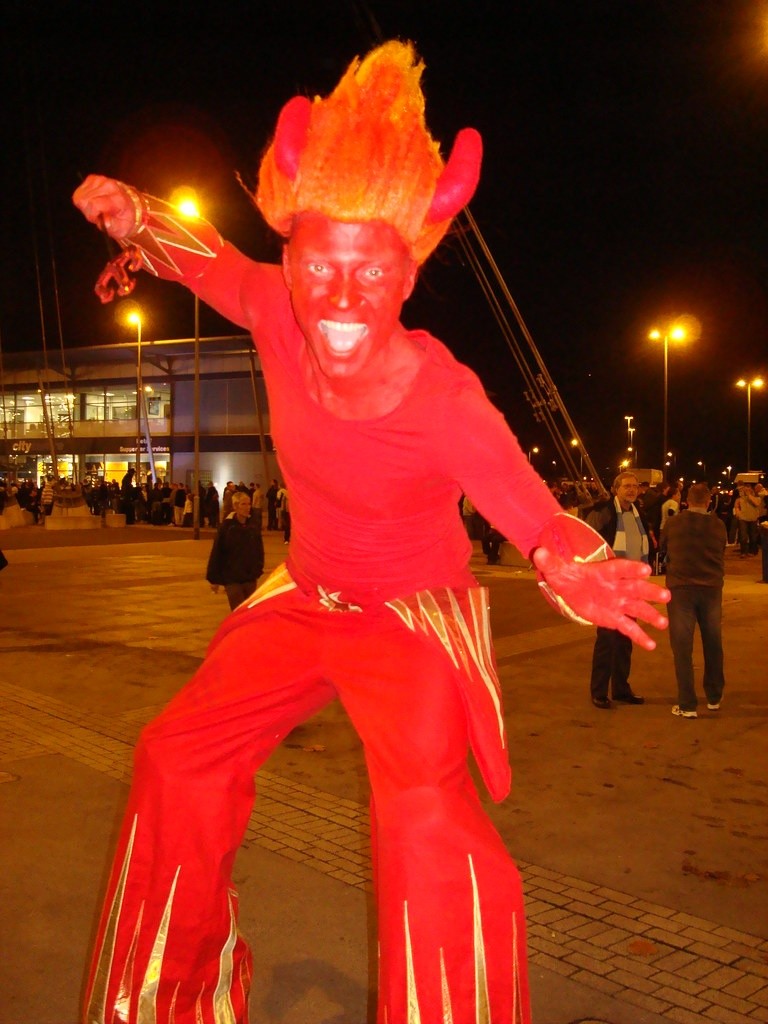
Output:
[
  {"xmin": 707, "ymin": 691, "xmax": 724, "ymax": 711},
  {"xmin": 671, "ymin": 705, "xmax": 698, "ymax": 719},
  {"xmin": 611, "ymin": 694, "xmax": 645, "ymax": 704},
  {"xmin": 591, "ymin": 696, "xmax": 610, "ymax": 708}
]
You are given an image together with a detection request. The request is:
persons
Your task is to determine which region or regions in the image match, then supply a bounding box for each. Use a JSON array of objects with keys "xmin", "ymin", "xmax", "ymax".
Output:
[
  {"xmin": 0, "ymin": 468, "xmax": 293, "ymax": 545},
  {"xmin": 206, "ymin": 492, "xmax": 265, "ymax": 611},
  {"xmin": 586, "ymin": 472, "xmax": 653, "ymax": 708},
  {"xmin": 460, "ymin": 476, "xmax": 768, "ymax": 582},
  {"xmin": 658, "ymin": 484, "xmax": 727, "ymax": 718},
  {"xmin": 72, "ymin": 41, "xmax": 672, "ymax": 1024}
]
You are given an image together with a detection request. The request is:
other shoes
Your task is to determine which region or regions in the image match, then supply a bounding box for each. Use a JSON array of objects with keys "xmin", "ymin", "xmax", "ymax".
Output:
[{"xmin": 284, "ymin": 540, "xmax": 289, "ymax": 545}]
